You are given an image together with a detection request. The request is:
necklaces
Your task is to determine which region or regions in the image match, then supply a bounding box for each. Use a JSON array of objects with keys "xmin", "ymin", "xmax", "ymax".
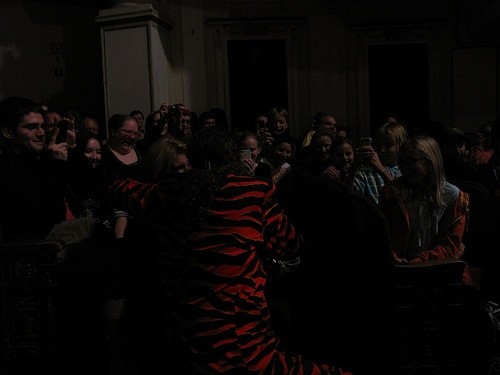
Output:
[{"xmin": 415, "ymin": 216, "xmax": 425, "ymax": 246}]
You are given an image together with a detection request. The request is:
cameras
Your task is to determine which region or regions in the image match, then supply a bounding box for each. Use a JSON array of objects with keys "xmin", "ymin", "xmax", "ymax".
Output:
[{"xmin": 165, "ymin": 104, "xmax": 179, "ymax": 114}]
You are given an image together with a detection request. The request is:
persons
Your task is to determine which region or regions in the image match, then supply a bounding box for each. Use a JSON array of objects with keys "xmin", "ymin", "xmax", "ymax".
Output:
[
  {"xmin": 69, "ymin": 132, "xmax": 127, "ymax": 239},
  {"xmin": 177, "ymin": 134, "xmax": 354, "ymax": 375},
  {"xmin": 1, "ymin": 97, "xmax": 69, "ymax": 235},
  {"xmin": 2, "ymin": 108, "xmax": 499, "ymax": 233},
  {"xmin": 370, "ymin": 136, "xmax": 468, "ymax": 265}
]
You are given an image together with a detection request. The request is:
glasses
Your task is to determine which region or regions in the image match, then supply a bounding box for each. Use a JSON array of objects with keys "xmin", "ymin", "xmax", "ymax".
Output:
[
  {"xmin": 119, "ymin": 129, "xmax": 139, "ymax": 138},
  {"xmin": 18, "ymin": 122, "xmax": 47, "ymax": 132},
  {"xmin": 376, "ymin": 142, "xmax": 394, "ymax": 150},
  {"xmin": 405, "ymin": 157, "xmax": 427, "ymax": 166},
  {"xmin": 182, "ymin": 120, "xmax": 192, "ymax": 125}
]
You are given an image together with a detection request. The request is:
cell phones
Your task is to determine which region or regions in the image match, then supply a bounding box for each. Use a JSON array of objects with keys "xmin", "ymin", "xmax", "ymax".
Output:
[
  {"xmin": 258, "ymin": 119, "xmax": 266, "ymax": 129},
  {"xmin": 240, "ymin": 149, "xmax": 251, "ymax": 162},
  {"xmin": 55, "ymin": 120, "xmax": 68, "ymax": 144},
  {"xmin": 359, "ymin": 137, "xmax": 372, "ymax": 152}
]
